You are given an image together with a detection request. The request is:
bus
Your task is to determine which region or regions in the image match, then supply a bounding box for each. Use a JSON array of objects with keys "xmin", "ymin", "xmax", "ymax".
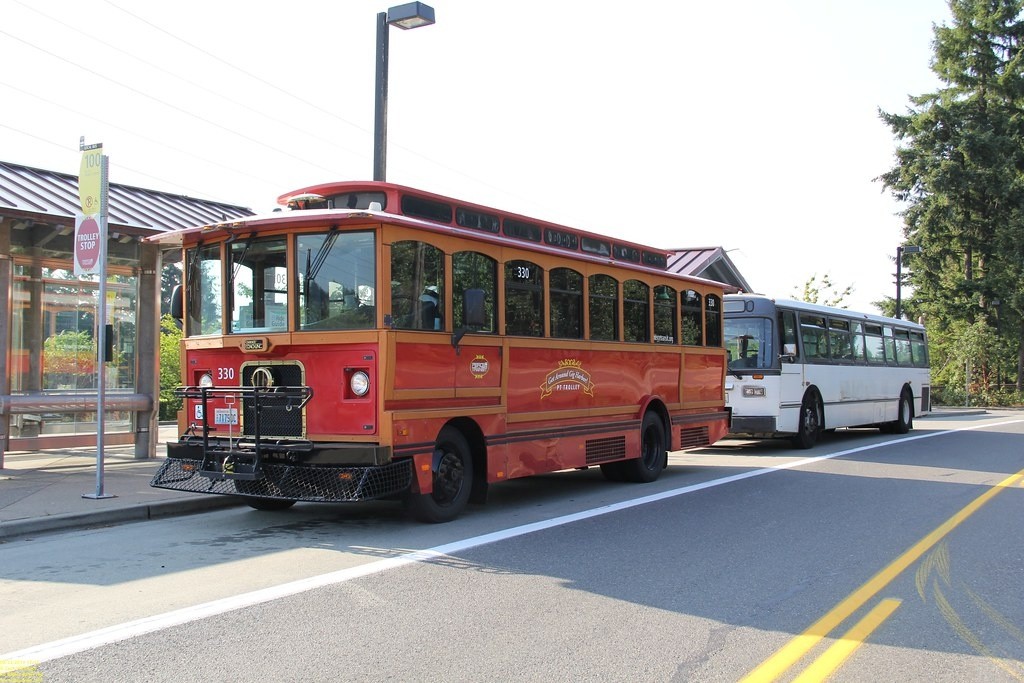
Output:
[
  {"xmin": 723, "ymin": 291, "xmax": 932, "ymax": 449},
  {"xmin": 0, "ymin": 253, "xmax": 156, "ymax": 427},
  {"xmin": 147, "ymin": 182, "xmax": 733, "ymax": 524}
]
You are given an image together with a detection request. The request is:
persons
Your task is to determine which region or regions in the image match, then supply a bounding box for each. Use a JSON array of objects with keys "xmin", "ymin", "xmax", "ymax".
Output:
[{"xmin": 395, "ymin": 261, "xmax": 441, "ymax": 329}]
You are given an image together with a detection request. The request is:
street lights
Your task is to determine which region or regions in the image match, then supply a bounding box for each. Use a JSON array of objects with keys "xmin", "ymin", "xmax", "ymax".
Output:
[
  {"xmin": 896, "ymin": 246, "xmax": 922, "ymax": 319},
  {"xmin": 991, "ymin": 300, "xmax": 1001, "ymax": 392},
  {"xmin": 374, "ymin": 1, "xmax": 436, "ymax": 182}
]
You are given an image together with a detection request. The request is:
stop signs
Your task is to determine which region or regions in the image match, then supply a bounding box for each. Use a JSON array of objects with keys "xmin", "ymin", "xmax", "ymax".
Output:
[{"xmin": 74, "ymin": 214, "xmax": 101, "ymax": 274}]
